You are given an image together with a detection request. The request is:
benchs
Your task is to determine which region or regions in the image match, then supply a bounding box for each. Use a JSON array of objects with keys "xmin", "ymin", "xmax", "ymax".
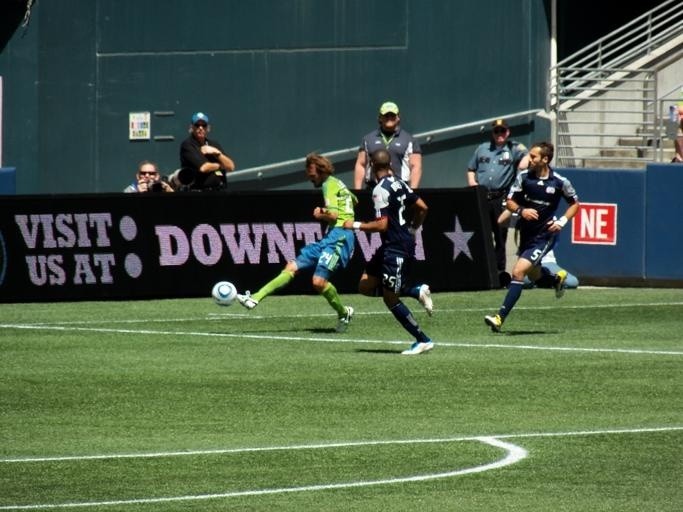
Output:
[{"xmin": 578, "ymin": 101, "xmax": 682, "ymax": 170}]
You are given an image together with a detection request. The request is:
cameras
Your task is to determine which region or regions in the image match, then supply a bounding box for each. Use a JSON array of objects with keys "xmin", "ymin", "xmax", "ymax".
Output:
[{"xmin": 147, "ymin": 180, "xmax": 163, "ymax": 192}]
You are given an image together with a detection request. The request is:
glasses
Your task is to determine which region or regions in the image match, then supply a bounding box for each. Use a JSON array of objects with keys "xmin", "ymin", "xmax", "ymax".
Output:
[{"xmin": 139, "ymin": 171, "xmax": 157, "ymax": 175}]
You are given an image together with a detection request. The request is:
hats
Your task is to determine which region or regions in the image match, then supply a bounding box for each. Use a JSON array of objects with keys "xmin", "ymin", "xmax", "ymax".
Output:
[
  {"xmin": 492, "ymin": 120, "xmax": 508, "ymax": 130},
  {"xmin": 380, "ymin": 102, "xmax": 399, "ymax": 116},
  {"xmin": 191, "ymin": 111, "xmax": 209, "ymax": 126}
]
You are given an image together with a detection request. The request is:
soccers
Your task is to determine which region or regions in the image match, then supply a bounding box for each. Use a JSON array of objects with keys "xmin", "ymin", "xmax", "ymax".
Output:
[{"xmin": 212, "ymin": 281, "xmax": 237, "ymax": 306}]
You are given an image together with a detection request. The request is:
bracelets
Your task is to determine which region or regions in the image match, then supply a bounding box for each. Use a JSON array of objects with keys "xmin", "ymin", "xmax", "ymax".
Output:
[
  {"xmin": 353, "ymin": 221, "xmax": 362, "ymax": 230},
  {"xmin": 216, "ymin": 151, "xmax": 222, "ymax": 158},
  {"xmin": 515, "ymin": 206, "xmax": 525, "ymax": 216},
  {"xmin": 559, "ymin": 215, "xmax": 568, "ymax": 225}
]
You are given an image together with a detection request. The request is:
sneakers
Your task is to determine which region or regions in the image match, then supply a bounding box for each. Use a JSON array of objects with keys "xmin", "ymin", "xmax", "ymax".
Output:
[
  {"xmin": 416, "ymin": 285, "xmax": 434, "ymax": 317},
  {"xmin": 484, "ymin": 314, "xmax": 504, "ymax": 332},
  {"xmin": 554, "ymin": 270, "xmax": 568, "ymax": 298},
  {"xmin": 336, "ymin": 306, "xmax": 354, "ymax": 332},
  {"xmin": 237, "ymin": 290, "xmax": 259, "ymax": 309},
  {"xmin": 401, "ymin": 337, "xmax": 434, "ymax": 355}
]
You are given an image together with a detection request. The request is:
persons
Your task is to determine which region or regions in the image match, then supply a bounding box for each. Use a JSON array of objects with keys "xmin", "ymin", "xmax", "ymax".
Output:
[
  {"xmin": 353, "ymin": 102, "xmax": 423, "ymax": 190},
  {"xmin": 671, "ymin": 119, "xmax": 683, "ymax": 163},
  {"xmin": 343, "ymin": 149, "xmax": 434, "ymax": 357},
  {"xmin": 484, "ymin": 141, "xmax": 579, "ymax": 334},
  {"xmin": 468, "ymin": 119, "xmax": 531, "ymax": 292},
  {"xmin": 122, "ymin": 160, "xmax": 176, "ymax": 193},
  {"xmin": 235, "ymin": 152, "xmax": 358, "ymax": 335},
  {"xmin": 179, "ymin": 112, "xmax": 235, "ymax": 192}
]
